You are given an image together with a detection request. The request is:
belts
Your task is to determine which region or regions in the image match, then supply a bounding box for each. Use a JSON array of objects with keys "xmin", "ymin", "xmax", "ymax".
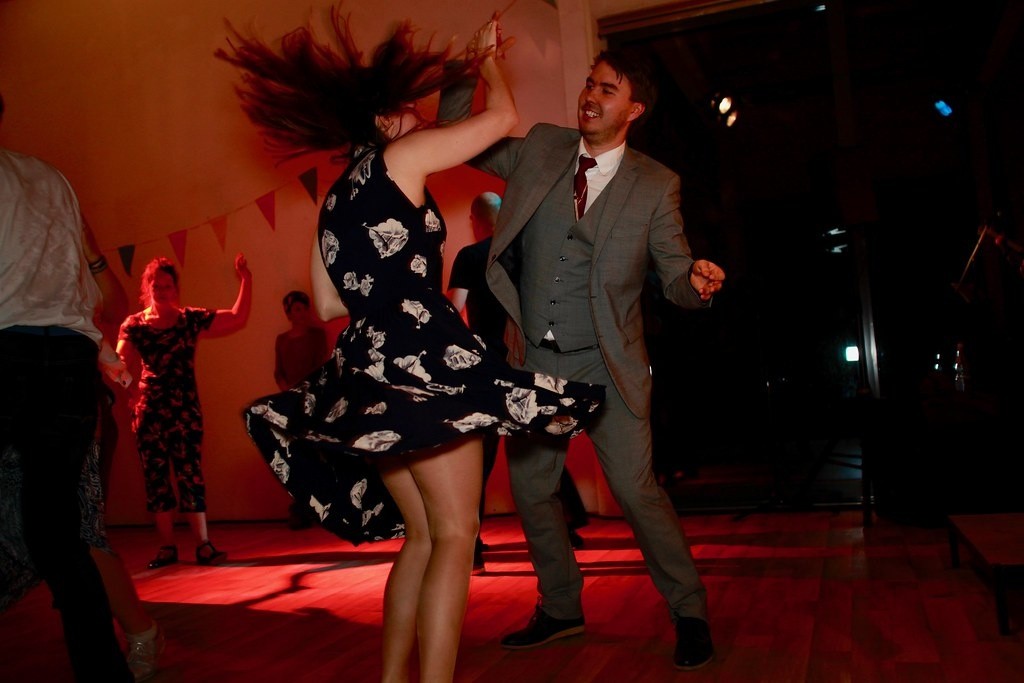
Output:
[{"xmin": 539, "ymin": 338, "xmax": 600, "ymax": 354}]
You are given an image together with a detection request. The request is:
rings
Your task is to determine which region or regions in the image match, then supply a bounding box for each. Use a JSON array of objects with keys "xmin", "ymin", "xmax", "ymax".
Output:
[{"xmin": 487, "ymin": 22, "xmax": 492, "ymax": 25}]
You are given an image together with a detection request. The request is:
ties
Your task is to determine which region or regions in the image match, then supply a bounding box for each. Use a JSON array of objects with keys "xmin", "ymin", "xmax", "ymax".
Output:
[{"xmin": 573, "ymin": 155, "xmax": 598, "ymax": 224}]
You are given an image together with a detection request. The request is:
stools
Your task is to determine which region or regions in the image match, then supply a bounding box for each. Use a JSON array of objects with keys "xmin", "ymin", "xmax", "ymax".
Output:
[{"xmin": 946, "ymin": 513, "xmax": 1023, "ymax": 636}]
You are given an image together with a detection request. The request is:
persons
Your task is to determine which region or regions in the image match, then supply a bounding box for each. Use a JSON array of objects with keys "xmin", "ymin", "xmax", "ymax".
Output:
[
  {"xmin": 116, "ymin": 252, "xmax": 252, "ymax": 569},
  {"xmin": 274, "ymin": 290, "xmax": 328, "ymax": 531},
  {"xmin": 215, "ymin": 20, "xmax": 607, "ymax": 683},
  {"xmin": 0, "ymin": 93, "xmax": 160, "ymax": 683},
  {"xmin": 436, "ymin": 10, "xmax": 725, "ymax": 670},
  {"xmin": 444, "ymin": 193, "xmax": 590, "ymax": 574}
]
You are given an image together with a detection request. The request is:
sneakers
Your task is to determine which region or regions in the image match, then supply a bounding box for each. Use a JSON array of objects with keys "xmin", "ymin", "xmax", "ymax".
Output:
[
  {"xmin": 672, "ymin": 617, "xmax": 715, "ymax": 672},
  {"xmin": 500, "ymin": 602, "xmax": 585, "ymax": 649}
]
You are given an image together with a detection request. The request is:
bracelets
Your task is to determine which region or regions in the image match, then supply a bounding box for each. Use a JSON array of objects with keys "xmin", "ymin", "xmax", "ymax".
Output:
[{"xmin": 89, "ymin": 255, "xmax": 109, "ymax": 275}]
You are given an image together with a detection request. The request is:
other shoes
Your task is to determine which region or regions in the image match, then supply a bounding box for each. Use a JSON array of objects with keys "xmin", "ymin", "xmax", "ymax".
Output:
[
  {"xmin": 289, "ymin": 514, "xmax": 313, "ymax": 531},
  {"xmin": 471, "ymin": 558, "xmax": 485, "ymax": 576},
  {"xmin": 147, "ymin": 543, "xmax": 178, "ymax": 570},
  {"xmin": 124, "ymin": 618, "xmax": 169, "ymax": 680},
  {"xmin": 568, "ymin": 528, "xmax": 586, "ymax": 549},
  {"xmin": 196, "ymin": 541, "xmax": 227, "ymax": 565}
]
